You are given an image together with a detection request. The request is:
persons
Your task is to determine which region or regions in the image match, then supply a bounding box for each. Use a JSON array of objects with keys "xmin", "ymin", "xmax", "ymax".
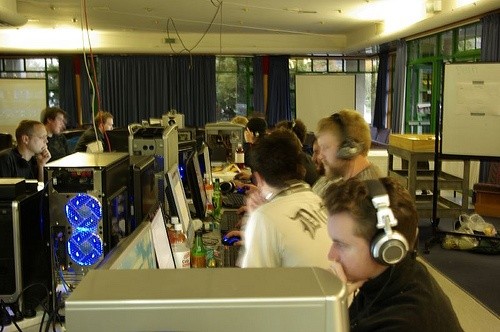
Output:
[
  {"xmin": 0, "ymin": 119, "xmax": 52, "ymax": 186},
  {"xmin": 73, "ymin": 111, "xmax": 113, "ymax": 153},
  {"xmin": 222, "ymin": 109, "xmax": 384, "ymax": 248},
  {"xmin": 323, "ymin": 177, "xmax": 464, "ymax": 332},
  {"xmin": 40, "ymin": 107, "xmax": 71, "ymax": 163},
  {"xmin": 233, "ymin": 128, "xmax": 359, "ymax": 309}
]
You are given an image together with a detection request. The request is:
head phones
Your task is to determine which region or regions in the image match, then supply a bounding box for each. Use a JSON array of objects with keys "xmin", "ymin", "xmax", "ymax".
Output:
[
  {"xmin": 364, "ymin": 179, "xmax": 409, "ymax": 266},
  {"xmin": 220, "ymin": 181, "xmax": 236, "ymax": 193},
  {"xmin": 329, "ymin": 113, "xmax": 362, "ymax": 161}
]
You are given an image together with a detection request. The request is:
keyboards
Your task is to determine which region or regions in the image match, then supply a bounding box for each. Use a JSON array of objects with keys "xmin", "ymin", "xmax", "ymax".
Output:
[
  {"xmin": 223, "ymin": 246, "xmax": 240, "ymax": 267},
  {"xmin": 220, "ymin": 214, "xmax": 243, "ymax": 232},
  {"xmin": 221, "ymin": 192, "xmax": 247, "ymax": 209}
]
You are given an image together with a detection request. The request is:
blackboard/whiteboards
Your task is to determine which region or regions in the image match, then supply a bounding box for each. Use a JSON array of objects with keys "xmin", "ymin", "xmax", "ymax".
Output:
[{"xmin": 442, "ymin": 62, "xmax": 500, "ymax": 158}]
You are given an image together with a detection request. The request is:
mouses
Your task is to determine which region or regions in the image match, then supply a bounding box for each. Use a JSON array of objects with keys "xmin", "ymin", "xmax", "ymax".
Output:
[
  {"xmin": 238, "ymin": 188, "xmax": 247, "ymax": 194},
  {"xmin": 222, "ymin": 235, "xmax": 240, "ymax": 247}
]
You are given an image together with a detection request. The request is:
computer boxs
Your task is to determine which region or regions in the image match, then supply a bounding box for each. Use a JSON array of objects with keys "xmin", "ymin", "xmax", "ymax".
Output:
[
  {"xmin": 206, "ymin": 123, "xmax": 246, "ymax": 164},
  {"xmin": 64, "ymin": 269, "xmax": 350, "ymax": 332},
  {"xmin": 0, "ymin": 126, "xmax": 196, "ymax": 317}
]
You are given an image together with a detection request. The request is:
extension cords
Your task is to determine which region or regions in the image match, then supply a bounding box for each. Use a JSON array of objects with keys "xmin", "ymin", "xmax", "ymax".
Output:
[{"xmin": 0, "ymin": 311, "xmax": 50, "ymax": 332}]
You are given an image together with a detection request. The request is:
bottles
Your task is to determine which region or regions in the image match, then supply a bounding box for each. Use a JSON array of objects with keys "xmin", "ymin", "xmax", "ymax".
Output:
[
  {"xmin": 204, "ymin": 173, "xmax": 212, "ymax": 203},
  {"xmin": 169, "ymin": 224, "xmax": 191, "ymax": 269},
  {"xmin": 212, "ymin": 178, "xmax": 221, "ymax": 207},
  {"xmin": 168, "ymin": 216, "xmax": 179, "ymax": 238},
  {"xmin": 235, "ymin": 143, "xmax": 244, "ymax": 168},
  {"xmin": 192, "ymin": 230, "xmax": 205, "ymax": 269}
]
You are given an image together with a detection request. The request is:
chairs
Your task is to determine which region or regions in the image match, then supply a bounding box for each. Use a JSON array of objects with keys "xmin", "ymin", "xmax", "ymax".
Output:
[
  {"xmin": 63, "ymin": 130, "xmax": 84, "ymax": 153},
  {"xmin": 0, "ymin": 134, "xmax": 12, "ymax": 151},
  {"xmin": 369, "ymin": 127, "xmax": 390, "ymax": 150}
]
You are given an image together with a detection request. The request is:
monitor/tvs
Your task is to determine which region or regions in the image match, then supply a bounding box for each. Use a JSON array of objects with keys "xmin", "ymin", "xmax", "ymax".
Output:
[{"xmin": 100, "ymin": 144, "xmax": 213, "ymax": 269}]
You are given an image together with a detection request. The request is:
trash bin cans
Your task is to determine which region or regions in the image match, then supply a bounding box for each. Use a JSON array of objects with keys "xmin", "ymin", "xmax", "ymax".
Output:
[{"xmin": 472, "ymin": 183, "xmax": 500, "ymax": 216}]
